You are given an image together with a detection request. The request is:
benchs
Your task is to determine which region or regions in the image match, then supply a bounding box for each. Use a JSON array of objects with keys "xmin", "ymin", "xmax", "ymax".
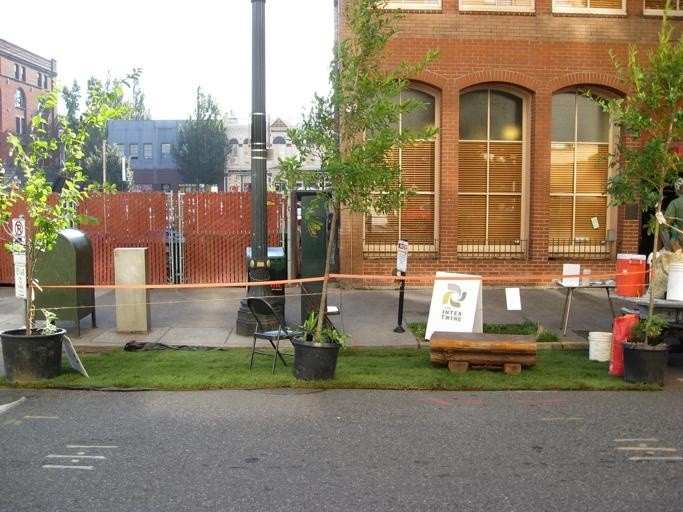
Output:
[{"xmin": 428, "ymin": 330, "xmax": 536, "ymax": 375}]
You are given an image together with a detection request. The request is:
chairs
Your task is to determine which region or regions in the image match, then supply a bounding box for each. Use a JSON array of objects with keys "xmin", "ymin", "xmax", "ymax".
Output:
[{"xmin": 245, "ymin": 296, "xmax": 302, "ymax": 374}]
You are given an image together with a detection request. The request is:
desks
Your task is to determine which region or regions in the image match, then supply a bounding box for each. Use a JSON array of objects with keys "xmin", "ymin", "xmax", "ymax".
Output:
[{"xmin": 554, "ymin": 279, "xmax": 615, "ymax": 336}]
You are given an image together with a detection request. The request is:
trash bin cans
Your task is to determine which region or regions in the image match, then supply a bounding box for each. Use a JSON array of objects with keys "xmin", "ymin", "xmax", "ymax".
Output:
[
  {"xmin": 27, "ymin": 228, "xmax": 97, "ymax": 337},
  {"xmin": 246, "ymin": 246, "xmax": 286, "ymax": 299}
]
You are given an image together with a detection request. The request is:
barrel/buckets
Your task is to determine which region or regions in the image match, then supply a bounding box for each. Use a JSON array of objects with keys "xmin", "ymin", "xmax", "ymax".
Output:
[
  {"xmin": 588, "ymin": 330, "xmax": 613, "ymax": 362},
  {"xmin": 667, "ymin": 263, "xmax": 682, "ymax": 301},
  {"xmin": 615, "ymin": 252, "xmax": 647, "ymax": 297}
]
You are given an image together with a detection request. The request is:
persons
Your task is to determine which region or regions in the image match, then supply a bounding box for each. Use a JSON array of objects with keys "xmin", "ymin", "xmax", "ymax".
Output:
[{"xmin": 661, "ymin": 178, "xmax": 682, "ymax": 252}]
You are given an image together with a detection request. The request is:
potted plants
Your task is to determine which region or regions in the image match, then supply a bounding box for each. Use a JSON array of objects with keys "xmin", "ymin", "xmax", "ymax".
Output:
[
  {"xmin": 576, "ymin": 1, "xmax": 682, "ymax": 386},
  {"xmin": 0, "ymin": 67, "xmax": 142, "ymax": 379},
  {"xmin": 267, "ymin": 1, "xmax": 440, "ymax": 382}
]
